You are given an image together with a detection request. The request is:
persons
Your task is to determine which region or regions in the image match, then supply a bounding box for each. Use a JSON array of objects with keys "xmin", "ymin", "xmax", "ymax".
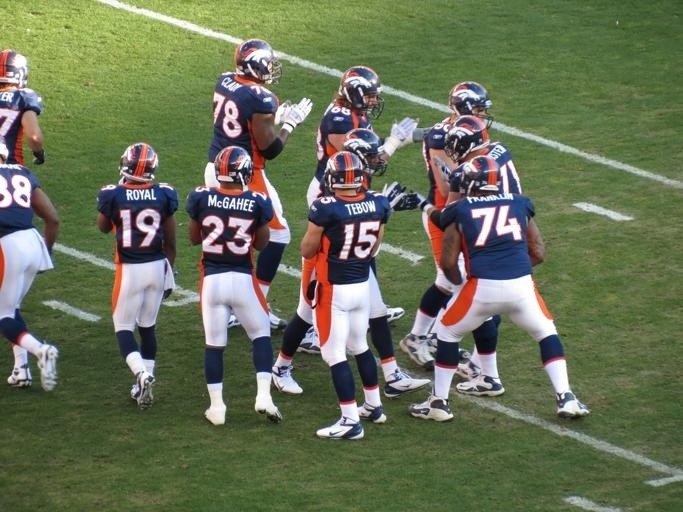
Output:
[
  {"xmin": 400, "ymin": 81, "xmax": 493, "ymax": 372},
  {"xmin": 407, "ymin": 155, "xmax": 591, "ymax": 423},
  {"xmin": 416, "ymin": 116, "xmax": 547, "ymax": 396},
  {"xmin": 207, "ymin": 39, "xmax": 313, "ymax": 329},
  {"xmin": 269, "ymin": 128, "xmax": 431, "ymax": 399},
  {"xmin": 0, "ymin": 145, "xmax": 60, "ymax": 393},
  {"xmin": 186, "ymin": 147, "xmax": 282, "ymax": 426},
  {"xmin": 96, "ymin": 143, "xmax": 179, "ymax": 411},
  {"xmin": 297, "ymin": 63, "xmax": 418, "ymax": 355},
  {"xmin": 300, "ymin": 151, "xmax": 390, "ymax": 440},
  {"xmin": 0, "ymin": 47, "xmax": 45, "ymax": 165}
]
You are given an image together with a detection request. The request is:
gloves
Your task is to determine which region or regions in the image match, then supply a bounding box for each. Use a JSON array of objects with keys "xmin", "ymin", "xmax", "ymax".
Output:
[
  {"xmin": 394, "ymin": 195, "xmax": 420, "ymax": 210},
  {"xmin": 32, "ymin": 149, "xmax": 46, "ymax": 165}
]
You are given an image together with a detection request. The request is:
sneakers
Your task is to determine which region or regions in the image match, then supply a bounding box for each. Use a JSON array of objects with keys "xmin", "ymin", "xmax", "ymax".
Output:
[
  {"xmin": 37, "ymin": 344, "xmax": 59, "ymax": 392},
  {"xmin": 7, "ymin": 364, "xmax": 32, "ymax": 388}
]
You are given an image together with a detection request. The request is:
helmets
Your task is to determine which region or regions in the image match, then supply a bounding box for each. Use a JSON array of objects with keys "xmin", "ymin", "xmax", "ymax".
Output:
[{"xmin": 1, "ymin": 49, "xmax": 29, "ymax": 89}]
